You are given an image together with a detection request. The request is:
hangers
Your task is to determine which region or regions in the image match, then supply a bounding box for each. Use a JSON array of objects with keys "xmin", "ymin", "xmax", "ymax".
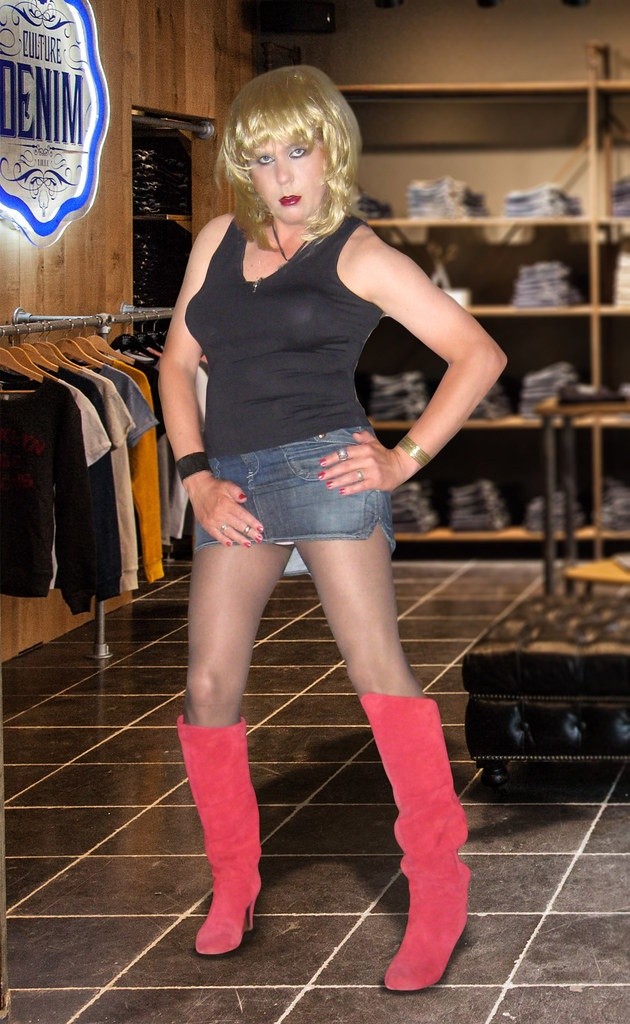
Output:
[{"xmin": 0, "ymin": 307, "xmax": 174, "ymax": 400}]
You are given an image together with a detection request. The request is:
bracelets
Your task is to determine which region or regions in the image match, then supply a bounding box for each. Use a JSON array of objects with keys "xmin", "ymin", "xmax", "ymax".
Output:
[
  {"xmin": 176, "ymin": 451, "xmax": 212, "ymax": 484},
  {"xmin": 398, "ymin": 436, "xmax": 431, "ymax": 466}
]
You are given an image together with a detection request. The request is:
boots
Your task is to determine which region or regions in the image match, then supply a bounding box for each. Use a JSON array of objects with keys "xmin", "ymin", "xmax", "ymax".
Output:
[
  {"xmin": 177, "ymin": 713, "xmax": 260, "ymax": 956},
  {"xmin": 357, "ymin": 692, "xmax": 472, "ymax": 993}
]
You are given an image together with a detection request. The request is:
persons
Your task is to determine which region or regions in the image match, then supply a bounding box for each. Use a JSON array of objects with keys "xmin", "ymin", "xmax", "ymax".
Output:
[{"xmin": 157, "ymin": 68, "xmax": 507, "ymax": 991}]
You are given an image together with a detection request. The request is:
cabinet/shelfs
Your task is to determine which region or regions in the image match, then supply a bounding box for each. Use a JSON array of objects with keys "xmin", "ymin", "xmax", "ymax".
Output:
[{"xmin": 331, "ymin": 79, "xmax": 630, "ymax": 542}]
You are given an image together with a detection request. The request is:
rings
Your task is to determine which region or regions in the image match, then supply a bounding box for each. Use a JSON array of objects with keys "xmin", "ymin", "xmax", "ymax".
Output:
[
  {"xmin": 221, "ymin": 524, "xmax": 229, "ymax": 532},
  {"xmin": 337, "ymin": 448, "xmax": 348, "ymax": 461},
  {"xmin": 243, "ymin": 525, "xmax": 251, "ymax": 536},
  {"xmin": 357, "ymin": 470, "xmax": 363, "ymax": 479}
]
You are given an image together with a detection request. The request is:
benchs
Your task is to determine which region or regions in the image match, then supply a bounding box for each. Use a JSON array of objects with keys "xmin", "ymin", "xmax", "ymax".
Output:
[{"xmin": 462, "ymin": 593, "xmax": 630, "ymax": 784}]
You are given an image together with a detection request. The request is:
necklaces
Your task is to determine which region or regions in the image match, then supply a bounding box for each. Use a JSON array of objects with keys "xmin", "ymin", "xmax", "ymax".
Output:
[{"xmin": 272, "ymin": 223, "xmax": 308, "ymax": 273}]
